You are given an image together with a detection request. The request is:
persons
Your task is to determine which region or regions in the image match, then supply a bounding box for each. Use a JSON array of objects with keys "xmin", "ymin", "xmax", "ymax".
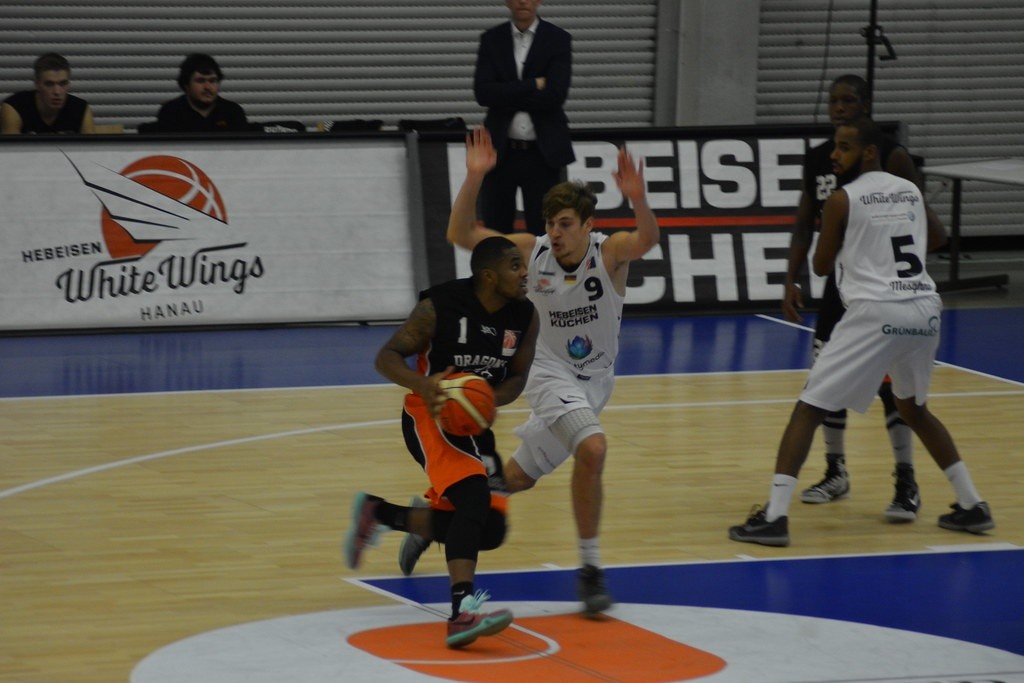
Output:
[
  {"xmin": 782, "ymin": 74, "xmax": 921, "ymax": 524},
  {"xmin": 729, "ymin": 121, "xmax": 995, "ymax": 549},
  {"xmin": 157, "ymin": 53, "xmax": 248, "ymax": 133},
  {"xmin": 399, "ymin": 125, "xmax": 660, "ymax": 619},
  {"xmin": 0, "ymin": 53, "xmax": 93, "ymax": 137},
  {"xmin": 345, "ymin": 237, "xmax": 541, "ymax": 649},
  {"xmin": 472, "ymin": 1, "xmax": 576, "ymax": 236}
]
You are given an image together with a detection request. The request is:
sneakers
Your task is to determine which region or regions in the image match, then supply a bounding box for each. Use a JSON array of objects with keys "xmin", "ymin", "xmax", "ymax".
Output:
[
  {"xmin": 399, "ymin": 495, "xmax": 441, "ymax": 576},
  {"xmin": 342, "ymin": 492, "xmax": 391, "ymax": 569},
  {"xmin": 446, "ymin": 589, "xmax": 512, "ymax": 648},
  {"xmin": 884, "ymin": 473, "xmax": 921, "ymax": 519},
  {"xmin": 728, "ymin": 502, "xmax": 791, "ymax": 546},
  {"xmin": 938, "ymin": 501, "xmax": 995, "ymax": 532},
  {"xmin": 800, "ymin": 470, "xmax": 850, "ymax": 503},
  {"xmin": 576, "ymin": 564, "xmax": 612, "ymax": 612}
]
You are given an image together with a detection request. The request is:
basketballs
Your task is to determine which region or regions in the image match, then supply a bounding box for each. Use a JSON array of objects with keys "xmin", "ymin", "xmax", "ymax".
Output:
[{"xmin": 437, "ymin": 372, "xmax": 496, "ymax": 436}]
